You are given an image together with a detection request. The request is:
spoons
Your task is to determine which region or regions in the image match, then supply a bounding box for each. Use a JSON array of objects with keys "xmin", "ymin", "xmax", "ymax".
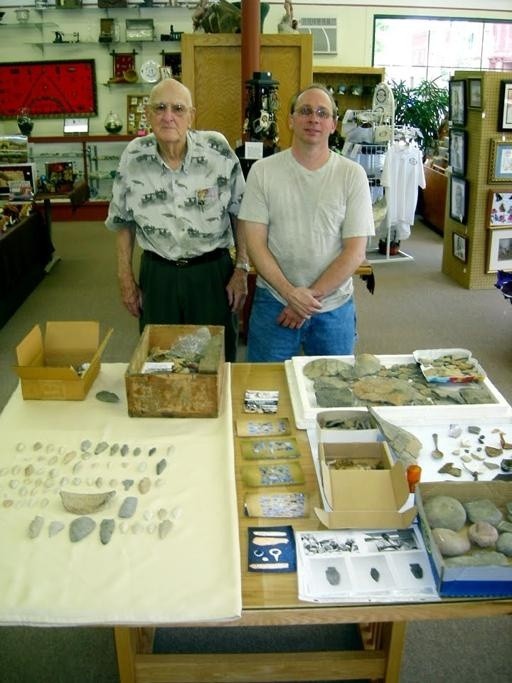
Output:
[{"xmin": 431, "ymin": 433, "xmax": 443, "ymax": 462}]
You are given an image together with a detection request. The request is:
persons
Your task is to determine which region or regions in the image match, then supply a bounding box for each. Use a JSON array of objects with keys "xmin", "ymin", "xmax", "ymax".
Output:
[
  {"xmin": 103, "ymin": 78, "xmax": 251, "ymax": 362},
  {"xmin": 238, "ymin": 84, "xmax": 376, "ymax": 363}
]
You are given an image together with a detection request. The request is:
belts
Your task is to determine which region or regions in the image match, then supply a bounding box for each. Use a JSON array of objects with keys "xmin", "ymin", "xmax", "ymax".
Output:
[{"xmin": 144, "ymin": 248, "xmax": 226, "ymax": 267}]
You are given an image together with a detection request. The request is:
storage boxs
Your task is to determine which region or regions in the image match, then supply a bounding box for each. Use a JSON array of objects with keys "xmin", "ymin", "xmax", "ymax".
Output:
[
  {"xmin": 130, "ymin": 324, "xmax": 227, "ymax": 420},
  {"xmin": 16, "ymin": 318, "xmax": 116, "ymax": 402}
]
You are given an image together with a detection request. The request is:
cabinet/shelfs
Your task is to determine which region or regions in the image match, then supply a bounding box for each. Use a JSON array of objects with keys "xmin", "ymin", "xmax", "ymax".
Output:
[
  {"xmin": 447, "ymin": 129, "xmax": 468, "ymax": 174},
  {"xmin": 183, "ymin": 33, "xmax": 313, "ymax": 162},
  {"xmin": 0, "ymin": 162, "xmax": 63, "ymax": 334},
  {"xmin": 29, "ymin": 136, "xmax": 147, "ymax": 222},
  {"xmin": 447, "ymin": 78, "xmax": 466, "ymax": 127},
  {"xmin": 311, "ymin": 65, "xmax": 387, "ymax": 152}
]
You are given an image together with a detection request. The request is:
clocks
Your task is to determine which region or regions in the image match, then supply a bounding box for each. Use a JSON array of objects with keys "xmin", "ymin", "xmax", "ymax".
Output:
[
  {"xmin": 110, "ymin": 49, "xmax": 138, "ymax": 83},
  {"xmin": 141, "ymin": 57, "xmax": 160, "ymax": 85}
]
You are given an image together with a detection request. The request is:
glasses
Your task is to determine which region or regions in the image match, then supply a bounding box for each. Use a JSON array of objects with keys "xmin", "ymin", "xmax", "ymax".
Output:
[
  {"xmin": 150, "ymin": 102, "xmax": 191, "ymax": 117},
  {"xmin": 292, "ymin": 106, "xmax": 337, "ymax": 120}
]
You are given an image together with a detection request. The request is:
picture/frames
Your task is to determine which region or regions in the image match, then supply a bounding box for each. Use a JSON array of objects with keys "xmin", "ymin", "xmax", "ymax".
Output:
[
  {"xmin": 449, "ymin": 179, "xmax": 467, "ymax": 222},
  {"xmin": 486, "ymin": 229, "xmax": 511, "ymax": 274},
  {"xmin": 467, "ymin": 75, "xmax": 483, "ymax": 112},
  {"xmin": 487, "ymin": 140, "xmax": 512, "ymax": 186},
  {"xmin": 449, "ymin": 233, "xmax": 468, "ymax": 260},
  {"xmin": 486, "ymin": 189, "xmax": 512, "ymax": 225},
  {"xmin": 496, "ymin": 82, "xmax": 512, "ymax": 134}
]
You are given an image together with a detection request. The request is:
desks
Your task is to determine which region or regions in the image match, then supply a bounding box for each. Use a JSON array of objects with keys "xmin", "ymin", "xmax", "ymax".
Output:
[{"xmin": 1, "ymin": 363, "xmax": 510, "ymax": 683}]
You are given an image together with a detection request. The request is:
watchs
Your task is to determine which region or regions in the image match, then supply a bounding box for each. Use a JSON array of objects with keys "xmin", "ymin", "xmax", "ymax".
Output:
[{"xmin": 235, "ymin": 263, "xmax": 249, "ymax": 271}]
